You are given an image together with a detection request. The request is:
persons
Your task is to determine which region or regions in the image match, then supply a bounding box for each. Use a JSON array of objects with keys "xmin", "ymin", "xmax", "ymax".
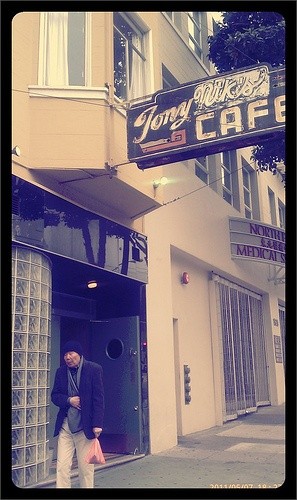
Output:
[{"xmin": 50, "ymin": 340, "xmax": 107, "ymax": 489}]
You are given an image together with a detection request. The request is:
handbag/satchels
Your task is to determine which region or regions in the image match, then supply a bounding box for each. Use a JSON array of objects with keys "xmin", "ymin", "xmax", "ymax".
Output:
[{"xmin": 84, "ymin": 437, "xmax": 105, "ymax": 465}]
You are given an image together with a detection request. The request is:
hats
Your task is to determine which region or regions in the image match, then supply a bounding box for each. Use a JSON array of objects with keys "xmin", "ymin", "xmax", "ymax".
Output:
[{"xmin": 62, "ymin": 340, "xmax": 83, "ymax": 358}]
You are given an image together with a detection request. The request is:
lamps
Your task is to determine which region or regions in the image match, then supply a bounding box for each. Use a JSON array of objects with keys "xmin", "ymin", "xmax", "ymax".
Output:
[
  {"xmin": 153, "ymin": 176, "xmax": 168, "ymax": 189},
  {"xmin": 87, "ymin": 279, "xmax": 97, "ymax": 288},
  {"xmin": 12, "ymin": 145, "xmax": 21, "ymax": 156}
]
